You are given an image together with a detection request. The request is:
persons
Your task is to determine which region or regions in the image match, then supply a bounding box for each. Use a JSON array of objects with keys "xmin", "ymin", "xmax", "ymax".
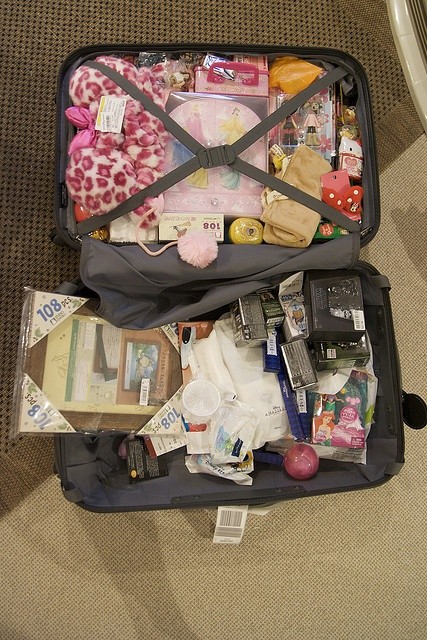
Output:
[{"xmin": 275, "ymin": 94, "xmax": 324, "ymax": 148}]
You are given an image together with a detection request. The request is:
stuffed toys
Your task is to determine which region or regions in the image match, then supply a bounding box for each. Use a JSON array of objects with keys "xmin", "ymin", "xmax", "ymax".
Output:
[{"xmin": 339, "ymin": 104, "xmax": 363, "ymax": 177}]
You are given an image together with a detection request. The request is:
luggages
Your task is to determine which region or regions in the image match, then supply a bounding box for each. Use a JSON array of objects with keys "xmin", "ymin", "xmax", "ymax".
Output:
[{"xmin": 50, "ymin": 44, "xmax": 427, "ymax": 514}]
